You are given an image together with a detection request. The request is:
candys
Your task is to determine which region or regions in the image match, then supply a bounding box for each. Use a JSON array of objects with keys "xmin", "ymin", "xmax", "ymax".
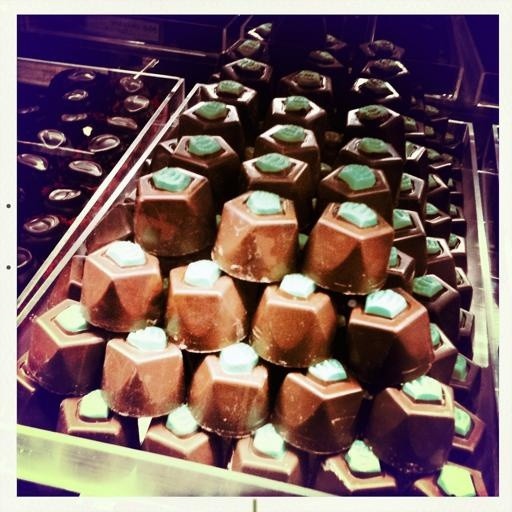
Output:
[{"xmin": 17, "ymin": 22, "xmax": 487, "ymax": 496}]
